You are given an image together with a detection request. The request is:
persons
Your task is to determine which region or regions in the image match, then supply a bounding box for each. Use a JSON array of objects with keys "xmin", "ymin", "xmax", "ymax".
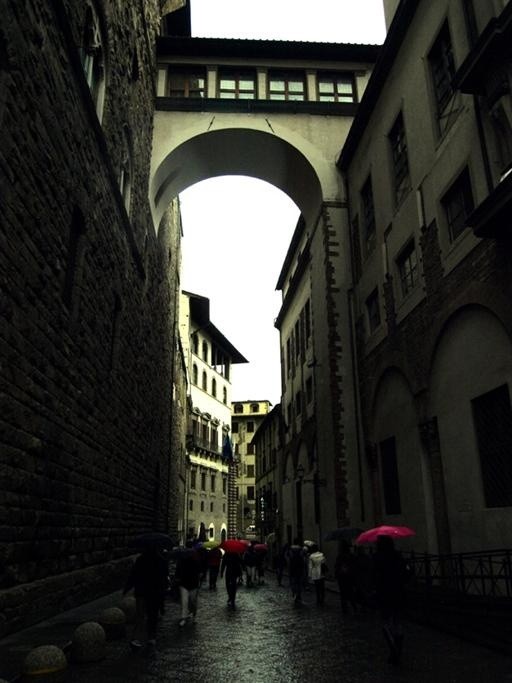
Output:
[
  {"xmin": 134, "ymin": 551, "xmax": 172, "ymax": 658},
  {"xmin": 272, "ymin": 551, "xmax": 287, "ymax": 585},
  {"xmin": 363, "ymin": 535, "xmax": 414, "ymax": 664},
  {"xmin": 307, "ymin": 545, "xmax": 326, "ymax": 603},
  {"xmin": 303, "ymin": 546, "xmax": 310, "ymax": 590},
  {"xmin": 186, "ymin": 527, "xmax": 197, "ymax": 547},
  {"xmin": 244, "ymin": 547, "xmax": 257, "ymax": 587},
  {"xmin": 221, "ymin": 551, "xmax": 247, "ymax": 606},
  {"xmin": 209, "ymin": 547, "xmax": 222, "ymax": 591},
  {"xmin": 285, "ymin": 537, "xmax": 309, "ymax": 600},
  {"xmin": 256, "ymin": 550, "xmax": 269, "ymax": 585},
  {"xmin": 335, "ymin": 539, "xmax": 362, "ymax": 627},
  {"xmin": 199, "ymin": 532, "xmax": 206, "ymax": 542},
  {"xmin": 173, "ymin": 542, "xmax": 205, "ymax": 627},
  {"xmin": 200, "ymin": 546, "xmax": 209, "ymax": 581}
]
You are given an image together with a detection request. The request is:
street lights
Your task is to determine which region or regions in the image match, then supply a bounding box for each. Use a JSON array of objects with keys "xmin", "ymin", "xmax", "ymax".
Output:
[{"xmin": 321, "ymin": 563, "xmax": 329, "ymax": 575}]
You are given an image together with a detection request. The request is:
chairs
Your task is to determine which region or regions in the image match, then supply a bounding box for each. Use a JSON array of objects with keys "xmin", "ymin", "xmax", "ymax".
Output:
[
  {"xmin": 193, "ymin": 616, "xmax": 197, "ymax": 622},
  {"xmin": 180, "ymin": 619, "xmax": 186, "ymax": 625},
  {"xmin": 131, "ymin": 640, "xmax": 142, "ymax": 646}
]
